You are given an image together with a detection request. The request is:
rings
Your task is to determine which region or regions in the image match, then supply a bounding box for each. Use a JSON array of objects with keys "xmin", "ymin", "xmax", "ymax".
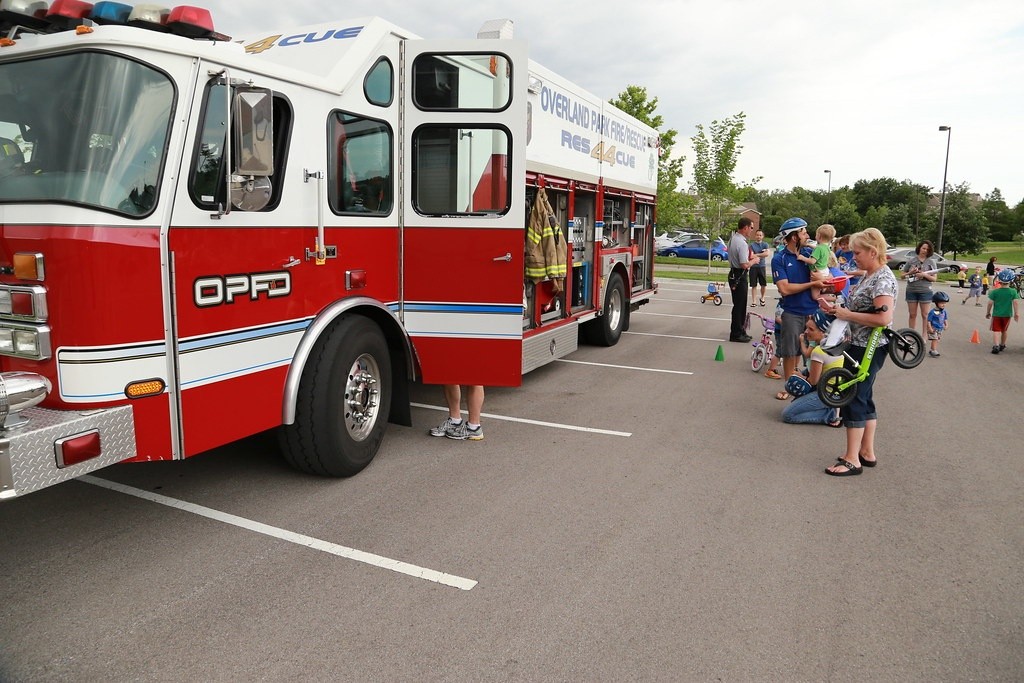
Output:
[
  {"xmin": 811, "ymin": 275, "xmax": 813, "ymax": 278},
  {"xmin": 834, "ymin": 314, "xmax": 836, "ymax": 316}
]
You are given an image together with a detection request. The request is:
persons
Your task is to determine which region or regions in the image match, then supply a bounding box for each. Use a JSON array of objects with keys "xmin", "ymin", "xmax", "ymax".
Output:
[
  {"xmin": 981, "ymin": 273, "xmax": 989, "ymax": 295},
  {"xmin": 749, "ymin": 230, "xmax": 770, "ymax": 307},
  {"xmin": 429, "ymin": 385, "xmax": 484, "ymax": 441},
  {"xmin": 728, "ymin": 217, "xmax": 760, "ymax": 343},
  {"xmin": 957, "ymin": 264, "xmax": 968, "ymax": 293},
  {"xmin": 241, "ymin": 175, "xmax": 256, "ymax": 210},
  {"xmin": 926, "ymin": 292, "xmax": 949, "ymax": 358},
  {"xmin": 986, "ymin": 257, "xmax": 998, "ymax": 286},
  {"xmin": 764, "ymin": 217, "xmax": 868, "ymax": 428},
  {"xmin": 961, "ymin": 266, "xmax": 984, "ymax": 307},
  {"xmin": 822, "ymin": 228, "xmax": 898, "ymax": 476},
  {"xmin": 986, "ymin": 269, "xmax": 1019, "ymax": 354},
  {"xmin": 901, "ymin": 240, "xmax": 937, "ymax": 350}
]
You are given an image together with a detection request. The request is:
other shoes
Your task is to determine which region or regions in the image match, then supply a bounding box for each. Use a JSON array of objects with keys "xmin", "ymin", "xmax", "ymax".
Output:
[
  {"xmin": 961, "ymin": 300, "xmax": 966, "ymax": 304},
  {"xmin": 975, "ymin": 303, "xmax": 982, "ymax": 306},
  {"xmin": 992, "ymin": 345, "xmax": 999, "ymax": 354},
  {"xmin": 929, "ymin": 349, "xmax": 940, "ymax": 356},
  {"xmin": 999, "ymin": 344, "xmax": 1005, "ymax": 351}
]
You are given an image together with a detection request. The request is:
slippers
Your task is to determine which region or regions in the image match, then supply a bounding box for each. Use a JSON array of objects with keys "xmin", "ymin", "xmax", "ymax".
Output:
[
  {"xmin": 775, "ymin": 391, "xmax": 790, "ymax": 400},
  {"xmin": 760, "ymin": 299, "xmax": 765, "ymax": 306},
  {"xmin": 751, "ymin": 303, "xmax": 757, "ymax": 307},
  {"xmin": 838, "ymin": 454, "xmax": 877, "ymax": 467},
  {"xmin": 830, "ymin": 411, "xmax": 843, "ymax": 428},
  {"xmin": 825, "ymin": 460, "xmax": 863, "ymax": 476}
]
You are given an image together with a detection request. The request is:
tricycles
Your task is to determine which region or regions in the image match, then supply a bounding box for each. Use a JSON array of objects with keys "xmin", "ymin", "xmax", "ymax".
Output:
[
  {"xmin": 748, "ymin": 312, "xmax": 783, "ymax": 372},
  {"xmin": 700, "ymin": 283, "xmax": 725, "ymax": 306}
]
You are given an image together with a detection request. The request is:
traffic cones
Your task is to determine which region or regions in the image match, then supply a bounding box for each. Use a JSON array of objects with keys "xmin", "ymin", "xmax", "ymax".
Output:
[
  {"xmin": 969, "ymin": 329, "xmax": 981, "ymax": 344},
  {"xmin": 714, "ymin": 345, "xmax": 724, "ymax": 361}
]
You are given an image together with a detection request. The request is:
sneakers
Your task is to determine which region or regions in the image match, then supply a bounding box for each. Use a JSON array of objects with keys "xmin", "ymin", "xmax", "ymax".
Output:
[
  {"xmin": 446, "ymin": 421, "xmax": 484, "ymax": 440},
  {"xmin": 429, "ymin": 417, "xmax": 463, "ymax": 436},
  {"xmin": 764, "ymin": 370, "xmax": 782, "ymax": 379}
]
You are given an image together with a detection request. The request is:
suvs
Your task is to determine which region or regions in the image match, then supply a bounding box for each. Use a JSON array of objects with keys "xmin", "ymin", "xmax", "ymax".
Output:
[
  {"xmin": 657, "ymin": 239, "xmax": 728, "ymax": 262},
  {"xmin": 654, "ymin": 230, "xmax": 726, "ymax": 253},
  {"xmin": 772, "ymin": 235, "xmax": 783, "ymax": 248}
]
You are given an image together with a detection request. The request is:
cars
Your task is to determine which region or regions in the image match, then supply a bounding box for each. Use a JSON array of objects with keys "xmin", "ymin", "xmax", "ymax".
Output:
[{"xmin": 886, "ymin": 248, "xmax": 968, "ymax": 274}]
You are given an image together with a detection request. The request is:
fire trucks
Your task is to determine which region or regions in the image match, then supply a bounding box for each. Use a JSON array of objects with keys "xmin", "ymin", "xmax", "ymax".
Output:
[{"xmin": 0, "ymin": 0, "xmax": 658, "ymax": 501}]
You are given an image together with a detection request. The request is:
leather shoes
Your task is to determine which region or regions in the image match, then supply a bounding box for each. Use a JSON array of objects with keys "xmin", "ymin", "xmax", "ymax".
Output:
[{"xmin": 730, "ymin": 335, "xmax": 752, "ymax": 343}]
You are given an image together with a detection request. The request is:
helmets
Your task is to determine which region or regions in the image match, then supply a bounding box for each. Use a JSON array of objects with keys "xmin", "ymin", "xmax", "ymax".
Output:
[
  {"xmin": 932, "ymin": 291, "xmax": 949, "ymax": 302},
  {"xmin": 825, "ymin": 275, "xmax": 855, "ymax": 292},
  {"xmin": 779, "ymin": 217, "xmax": 807, "ymax": 235},
  {"xmin": 785, "ymin": 375, "xmax": 812, "ymax": 396},
  {"xmin": 999, "ymin": 270, "xmax": 1015, "ymax": 283}
]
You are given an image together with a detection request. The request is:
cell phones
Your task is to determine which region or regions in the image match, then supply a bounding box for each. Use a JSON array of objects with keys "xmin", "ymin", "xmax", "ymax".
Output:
[{"xmin": 817, "ymin": 298, "xmax": 831, "ymax": 309}]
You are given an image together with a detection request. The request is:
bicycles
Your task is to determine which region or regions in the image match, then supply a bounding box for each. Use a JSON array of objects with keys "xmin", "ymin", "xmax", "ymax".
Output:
[
  {"xmin": 817, "ymin": 305, "xmax": 926, "ymax": 408},
  {"xmin": 992, "ymin": 267, "xmax": 1024, "ymax": 299}
]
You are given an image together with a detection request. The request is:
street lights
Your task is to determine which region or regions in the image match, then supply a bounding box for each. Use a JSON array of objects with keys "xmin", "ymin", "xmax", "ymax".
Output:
[
  {"xmin": 936, "ymin": 126, "xmax": 951, "ymax": 253},
  {"xmin": 824, "ymin": 170, "xmax": 831, "ymax": 224}
]
